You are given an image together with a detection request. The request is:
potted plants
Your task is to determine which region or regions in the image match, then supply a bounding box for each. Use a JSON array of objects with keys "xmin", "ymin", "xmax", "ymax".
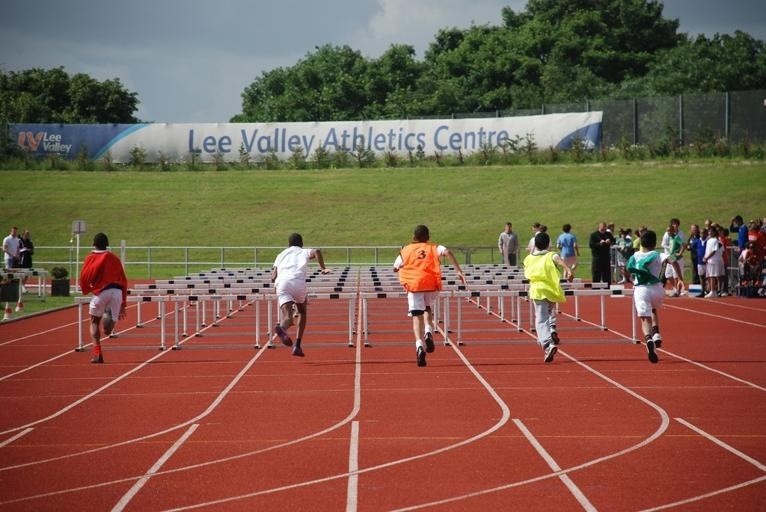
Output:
[{"xmin": 49, "ymin": 264, "xmax": 70, "ymax": 297}]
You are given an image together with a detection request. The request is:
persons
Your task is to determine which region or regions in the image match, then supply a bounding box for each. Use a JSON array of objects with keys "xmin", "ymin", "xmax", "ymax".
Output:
[
  {"xmin": 622, "ymin": 231, "xmax": 684, "ymax": 363},
  {"xmin": 522, "ymin": 233, "xmax": 573, "ymax": 362},
  {"xmin": 392, "ymin": 223, "xmax": 467, "ymax": 366},
  {"xmin": 16, "ymin": 231, "xmax": 33, "ymax": 294},
  {"xmin": 497, "ymin": 215, "xmax": 766, "ymax": 299},
  {"xmin": 269, "ymin": 233, "xmax": 329, "ymax": 357},
  {"xmin": 78, "ymin": 232, "xmax": 127, "ymax": 364},
  {"xmin": 1, "ymin": 226, "xmax": 24, "ymax": 269}
]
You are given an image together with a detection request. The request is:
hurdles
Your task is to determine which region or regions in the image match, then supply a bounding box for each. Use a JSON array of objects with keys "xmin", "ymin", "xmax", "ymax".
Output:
[
  {"xmin": 170, "ymin": 294, "xmax": 265, "ymax": 349},
  {"xmin": 292, "ymin": 261, "xmax": 412, "ymax": 334},
  {"xmin": 1, "ymin": 268, "xmax": 48, "ymax": 303},
  {"xmin": 360, "ymin": 292, "xmax": 453, "ymax": 347},
  {"xmin": 453, "ymin": 289, "xmax": 542, "ymax": 347},
  {"xmin": 359, "ymin": 286, "xmax": 439, "ymax": 333},
  {"xmin": 108, "ymin": 288, "xmax": 191, "ymax": 337},
  {"xmin": 265, "ymin": 292, "xmax": 358, "ymax": 348},
  {"xmin": 553, "ymin": 289, "xmax": 642, "ymax": 344},
  {"xmin": 126, "ymin": 268, "xmax": 280, "ymax": 336},
  {"xmin": 74, "ymin": 297, "xmax": 170, "ymax": 351},
  {"xmin": 306, "ymin": 286, "xmax": 359, "ymax": 334},
  {"xmin": 431, "ymin": 263, "xmax": 583, "ymax": 323},
  {"xmin": 190, "ymin": 287, "xmax": 276, "ymax": 337},
  {"xmin": 440, "ymin": 283, "xmax": 524, "ymax": 333},
  {"xmin": 525, "ymin": 283, "xmax": 609, "ymax": 332}
]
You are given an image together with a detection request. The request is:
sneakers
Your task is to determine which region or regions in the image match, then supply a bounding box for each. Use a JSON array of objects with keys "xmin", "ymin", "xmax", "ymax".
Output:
[
  {"xmin": 653, "ymin": 333, "xmax": 662, "ymax": 348},
  {"xmin": 291, "ymin": 346, "xmax": 304, "ymax": 355},
  {"xmin": 90, "ymin": 356, "xmax": 103, "ymax": 363},
  {"xmin": 417, "ymin": 346, "xmax": 426, "ymax": 366},
  {"xmin": 275, "ymin": 326, "xmax": 292, "ymax": 346},
  {"xmin": 551, "ymin": 329, "xmax": 559, "ymax": 344},
  {"xmin": 617, "ymin": 279, "xmax": 729, "ymax": 298},
  {"xmin": 646, "ymin": 339, "xmax": 659, "ymax": 363},
  {"xmin": 424, "ymin": 332, "xmax": 434, "ymax": 353},
  {"xmin": 102, "ymin": 308, "xmax": 111, "ymax": 328},
  {"xmin": 544, "ymin": 344, "xmax": 557, "ymax": 362}
]
